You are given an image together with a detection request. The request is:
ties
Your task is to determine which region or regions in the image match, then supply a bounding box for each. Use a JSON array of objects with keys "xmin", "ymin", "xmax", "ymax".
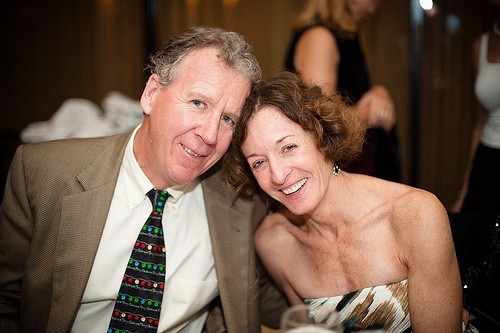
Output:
[{"xmin": 106, "ymin": 189, "xmax": 171, "ymax": 333}]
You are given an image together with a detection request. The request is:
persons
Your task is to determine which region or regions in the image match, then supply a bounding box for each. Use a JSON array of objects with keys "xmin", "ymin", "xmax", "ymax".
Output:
[
  {"xmin": 454, "ymin": 24, "xmax": 500, "ymax": 278},
  {"xmin": 1, "ymin": 22, "xmax": 293, "ymax": 332},
  {"xmin": 284, "ymin": 0, "xmax": 402, "ymax": 184},
  {"xmin": 221, "ymin": 70, "xmax": 482, "ymax": 333}
]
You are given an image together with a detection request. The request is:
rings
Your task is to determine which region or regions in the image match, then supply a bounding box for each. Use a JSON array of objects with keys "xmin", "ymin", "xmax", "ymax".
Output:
[{"xmin": 381, "ymin": 110, "xmax": 387, "ymax": 121}]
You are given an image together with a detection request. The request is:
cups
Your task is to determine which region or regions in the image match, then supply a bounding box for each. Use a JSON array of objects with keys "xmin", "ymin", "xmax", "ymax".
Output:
[{"xmin": 279, "ymin": 304, "xmax": 344, "ymax": 333}]
